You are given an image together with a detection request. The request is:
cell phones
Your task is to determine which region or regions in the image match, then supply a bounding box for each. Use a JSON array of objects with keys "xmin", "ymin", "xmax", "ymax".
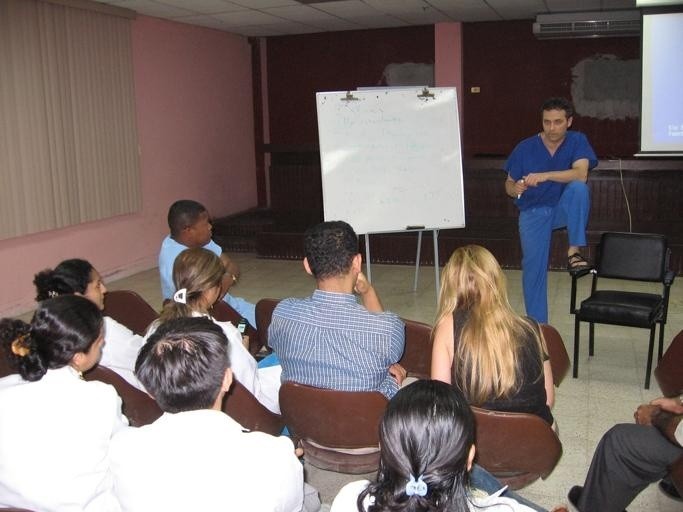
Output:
[{"xmin": 236, "ymin": 318, "xmax": 248, "ymax": 338}]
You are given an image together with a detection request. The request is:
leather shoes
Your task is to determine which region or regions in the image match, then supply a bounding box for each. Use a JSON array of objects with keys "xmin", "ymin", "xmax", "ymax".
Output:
[
  {"xmin": 659, "ymin": 480, "xmax": 683, "ymax": 502},
  {"xmin": 568, "ymin": 486, "xmax": 626, "ymax": 512}
]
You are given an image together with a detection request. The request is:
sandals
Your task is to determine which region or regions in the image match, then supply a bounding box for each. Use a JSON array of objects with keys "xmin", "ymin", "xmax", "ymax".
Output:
[{"xmin": 566, "ymin": 253, "xmax": 593, "ymax": 277}]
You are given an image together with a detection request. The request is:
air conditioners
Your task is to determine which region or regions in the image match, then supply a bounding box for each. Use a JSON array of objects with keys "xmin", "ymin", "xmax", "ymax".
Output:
[{"xmin": 532, "ymin": 9, "xmax": 640, "ymax": 40}]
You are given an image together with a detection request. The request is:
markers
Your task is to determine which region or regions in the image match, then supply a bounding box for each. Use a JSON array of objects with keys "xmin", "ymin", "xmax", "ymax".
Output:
[{"xmin": 518, "ymin": 179, "xmax": 525, "ymax": 200}]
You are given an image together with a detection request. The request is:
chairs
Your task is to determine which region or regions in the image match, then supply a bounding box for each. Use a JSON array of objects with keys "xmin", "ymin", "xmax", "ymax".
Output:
[
  {"xmin": 569, "ymin": 232, "xmax": 674, "ymax": 389},
  {"xmin": 83, "ymin": 288, "xmax": 568, "ymax": 492}
]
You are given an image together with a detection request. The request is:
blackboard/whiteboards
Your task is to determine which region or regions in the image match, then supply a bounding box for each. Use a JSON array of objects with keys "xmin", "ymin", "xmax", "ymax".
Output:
[{"xmin": 316, "ymin": 85, "xmax": 465, "ymax": 236}]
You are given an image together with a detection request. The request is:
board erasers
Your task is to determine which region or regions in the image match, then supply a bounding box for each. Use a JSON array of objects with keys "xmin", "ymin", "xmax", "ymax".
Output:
[{"xmin": 407, "ymin": 225, "xmax": 425, "ymax": 230}]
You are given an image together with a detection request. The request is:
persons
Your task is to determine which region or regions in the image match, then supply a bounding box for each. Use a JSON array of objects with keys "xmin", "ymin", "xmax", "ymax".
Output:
[
  {"xmin": 266, "ymin": 220, "xmax": 408, "ymax": 401},
  {"xmin": 431, "ymin": 244, "xmax": 560, "ymax": 436},
  {"xmin": 111, "ymin": 317, "xmax": 304, "ymax": 512},
  {"xmin": 158, "ymin": 200, "xmax": 257, "ymax": 329},
  {"xmin": 567, "ymin": 396, "xmax": 683, "ymax": 511},
  {"xmin": 331, "ymin": 379, "xmax": 538, "ymax": 512},
  {"xmin": 505, "ymin": 97, "xmax": 598, "ymax": 325},
  {"xmin": 32, "ymin": 258, "xmax": 147, "ymax": 389},
  {"xmin": 142, "ymin": 248, "xmax": 283, "ymax": 415},
  {"xmin": 0, "ymin": 295, "xmax": 130, "ymax": 512}
]
